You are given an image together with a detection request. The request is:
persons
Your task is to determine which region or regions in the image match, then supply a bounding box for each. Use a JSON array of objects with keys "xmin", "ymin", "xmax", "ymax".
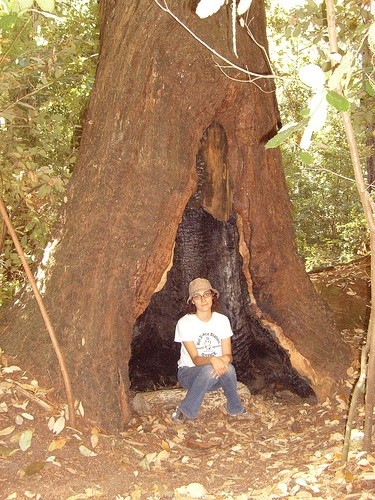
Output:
[{"xmin": 171, "ymin": 278, "xmax": 254, "ymax": 425}]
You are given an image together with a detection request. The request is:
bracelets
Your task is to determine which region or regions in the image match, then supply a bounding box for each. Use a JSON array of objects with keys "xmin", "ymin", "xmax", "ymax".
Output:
[{"xmin": 225, "ymin": 354, "xmax": 233, "ymax": 364}]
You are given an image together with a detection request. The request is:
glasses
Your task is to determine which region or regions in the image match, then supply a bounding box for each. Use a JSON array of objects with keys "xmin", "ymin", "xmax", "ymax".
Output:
[{"xmin": 193, "ymin": 293, "xmax": 212, "ymax": 300}]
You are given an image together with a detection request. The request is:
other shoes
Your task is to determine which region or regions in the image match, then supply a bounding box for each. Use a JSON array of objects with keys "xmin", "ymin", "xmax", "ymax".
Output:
[
  {"xmin": 171, "ymin": 408, "xmax": 186, "ymax": 423},
  {"xmin": 240, "ymin": 408, "xmax": 255, "ymax": 420}
]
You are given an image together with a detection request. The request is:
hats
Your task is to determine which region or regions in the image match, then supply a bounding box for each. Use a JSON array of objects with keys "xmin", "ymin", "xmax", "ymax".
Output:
[{"xmin": 187, "ymin": 278, "xmax": 220, "ymax": 305}]
